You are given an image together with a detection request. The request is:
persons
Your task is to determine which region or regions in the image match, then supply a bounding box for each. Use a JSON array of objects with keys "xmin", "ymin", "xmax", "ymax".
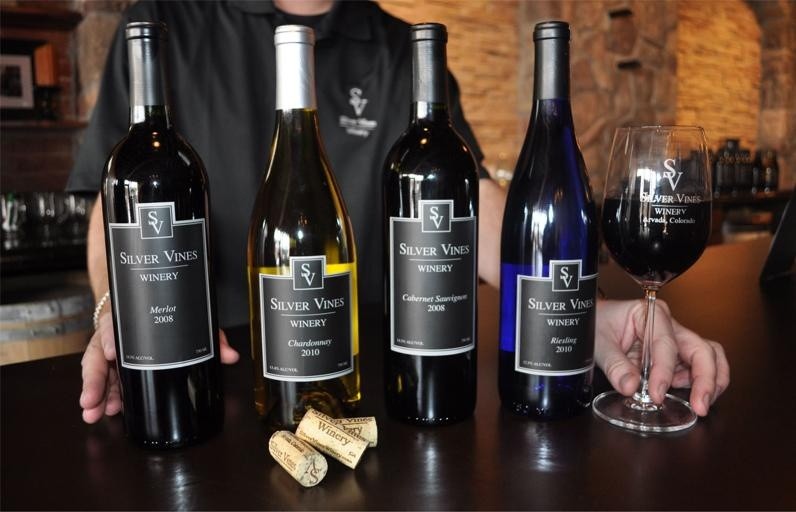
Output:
[{"xmin": 63, "ymin": 0, "xmax": 731, "ymax": 416}]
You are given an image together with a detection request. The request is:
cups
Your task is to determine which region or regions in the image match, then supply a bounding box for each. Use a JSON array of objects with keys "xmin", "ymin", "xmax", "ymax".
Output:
[{"xmin": 3, "ymin": 183, "xmax": 95, "ymax": 236}]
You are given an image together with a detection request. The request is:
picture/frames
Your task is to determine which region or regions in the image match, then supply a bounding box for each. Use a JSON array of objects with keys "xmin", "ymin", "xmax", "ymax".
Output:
[{"xmin": 0, "ymin": 51, "xmax": 37, "ymax": 114}]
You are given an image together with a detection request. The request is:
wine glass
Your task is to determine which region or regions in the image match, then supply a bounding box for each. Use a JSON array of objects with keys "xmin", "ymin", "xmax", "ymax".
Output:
[{"xmin": 591, "ymin": 125, "xmax": 716, "ymax": 434}]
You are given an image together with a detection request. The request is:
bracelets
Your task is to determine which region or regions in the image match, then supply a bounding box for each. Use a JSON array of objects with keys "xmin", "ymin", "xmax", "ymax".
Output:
[{"xmin": 93, "ymin": 291, "xmax": 111, "ymax": 330}]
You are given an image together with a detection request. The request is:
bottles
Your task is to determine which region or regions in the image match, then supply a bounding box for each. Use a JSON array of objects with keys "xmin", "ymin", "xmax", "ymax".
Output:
[
  {"xmin": 495, "ymin": 18, "xmax": 598, "ymax": 421},
  {"xmin": 701, "ymin": 133, "xmax": 781, "ymax": 196},
  {"xmin": 99, "ymin": 19, "xmax": 227, "ymax": 450},
  {"xmin": 379, "ymin": 22, "xmax": 480, "ymax": 428},
  {"xmin": 247, "ymin": 23, "xmax": 367, "ymax": 431}
]
